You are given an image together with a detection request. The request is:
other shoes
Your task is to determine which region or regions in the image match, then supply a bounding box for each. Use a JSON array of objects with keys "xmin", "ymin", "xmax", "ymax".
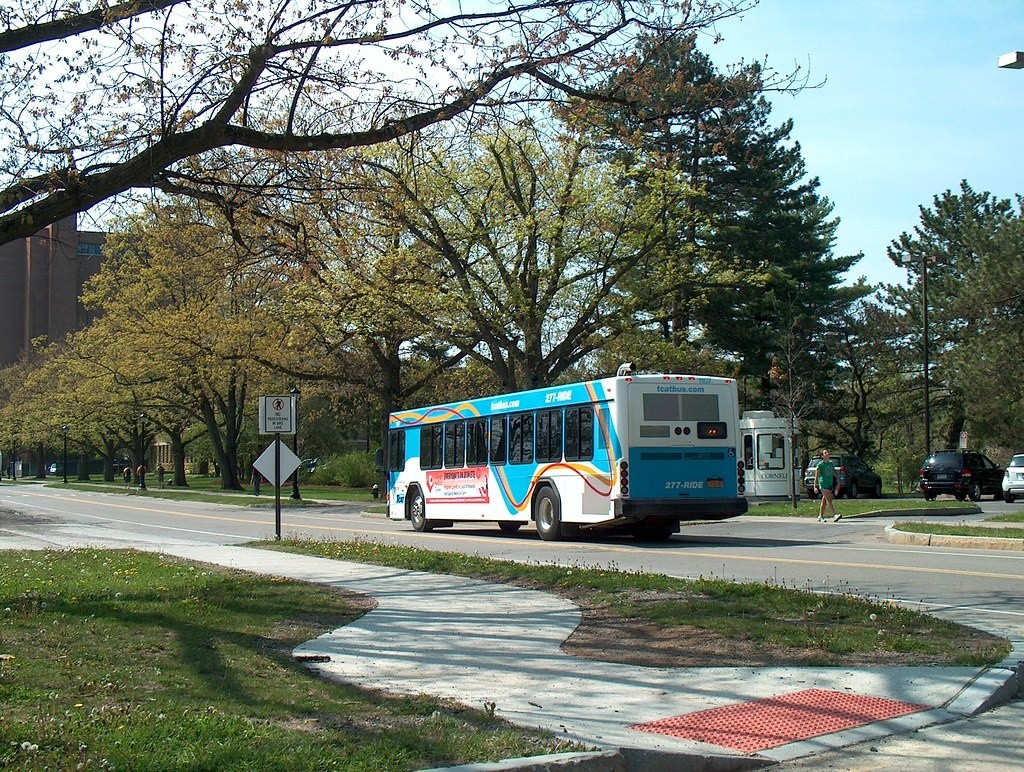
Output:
[
  {"xmin": 817, "ymin": 516, "xmax": 826, "ymax": 522},
  {"xmin": 834, "ymin": 514, "xmax": 842, "ymax": 522}
]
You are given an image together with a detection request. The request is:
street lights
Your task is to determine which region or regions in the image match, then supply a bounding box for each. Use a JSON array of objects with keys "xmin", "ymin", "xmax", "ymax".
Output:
[
  {"xmin": 138, "ymin": 410, "xmax": 147, "ymax": 490},
  {"xmin": 901, "ymin": 250, "xmax": 948, "ymax": 456},
  {"xmin": 62, "ymin": 425, "xmax": 68, "ymax": 483},
  {"xmin": 290, "ymin": 388, "xmax": 301, "ymax": 499}
]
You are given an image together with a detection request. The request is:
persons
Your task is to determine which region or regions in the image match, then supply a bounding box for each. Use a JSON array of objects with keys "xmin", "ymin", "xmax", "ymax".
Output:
[
  {"xmin": 813, "ymin": 450, "xmax": 842, "ymax": 522},
  {"xmin": 6, "ymin": 464, "xmax": 12, "ymax": 479},
  {"xmin": 123, "ymin": 464, "xmax": 131, "ymax": 489},
  {"xmin": 136, "ymin": 464, "xmax": 147, "ymax": 490},
  {"xmin": 156, "ymin": 462, "xmax": 164, "ymax": 489}
]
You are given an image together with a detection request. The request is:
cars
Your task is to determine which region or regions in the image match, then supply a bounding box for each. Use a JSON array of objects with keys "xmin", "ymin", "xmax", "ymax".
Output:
[
  {"xmin": 48, "ymin": 464, "xmax": 63, "ymax": 476},
  {"xmin": 113, "ymin": 464, "xmax": 126, "ymax": 476},
  {"xmin": 299, "ymin": 459, "xmax": 312, "ymax": 474},
  {"xmin": 1002, "ymin": 454, "xmax": 1024, "ymax": 504},
  {"xmin": 321, "ymin": 458, "xmax": 341, "ymax": 473},
  {"xmin": 307, "ymin": 457, "xmax": 327, "ymax": 474}
]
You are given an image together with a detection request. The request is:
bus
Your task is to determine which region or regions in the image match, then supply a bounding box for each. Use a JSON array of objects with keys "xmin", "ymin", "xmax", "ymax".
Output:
[{"xmin": 376, "ymin": 363, "xmax": 747, "ymax": 542}]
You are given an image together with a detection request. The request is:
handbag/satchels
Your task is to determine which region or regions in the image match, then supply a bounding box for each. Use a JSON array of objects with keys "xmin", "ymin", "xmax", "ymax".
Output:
[{"xmin": 833, "ymin": 476, "xmax": 838, "ymax": 489}]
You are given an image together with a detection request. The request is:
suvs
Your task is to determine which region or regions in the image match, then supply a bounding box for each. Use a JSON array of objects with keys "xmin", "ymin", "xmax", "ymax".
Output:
[
  {"xmin": 804, "ymin": 453, "xmax": 882, "ymax": 499},
  {"xmin": 919, "ymin": 449, "xmax": 1004, "ymax": 501}
]
